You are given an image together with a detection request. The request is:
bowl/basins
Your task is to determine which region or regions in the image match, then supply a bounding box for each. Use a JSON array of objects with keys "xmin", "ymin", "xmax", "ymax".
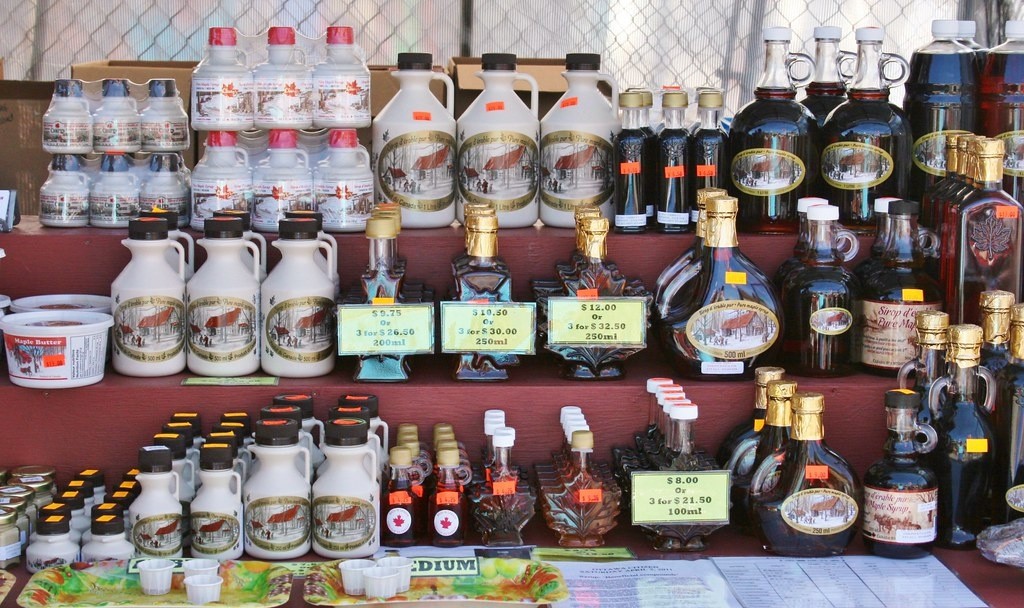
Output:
[{"xmin": 3, "ymin": 293, "xmax": 114, "ymax": 389}]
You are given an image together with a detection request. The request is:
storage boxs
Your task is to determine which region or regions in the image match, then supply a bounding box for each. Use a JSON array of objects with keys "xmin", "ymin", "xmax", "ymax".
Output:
[
  {"xmin": 358, "ymin": 63, "xmax": 445, "ymax": 170},
  {"xmin": 70, "ymin": 59, "xmax": 196, "ymax": 174},
  {"xmin": 447, "ymin": 56, "xmax": 612, "ymax": 121}
]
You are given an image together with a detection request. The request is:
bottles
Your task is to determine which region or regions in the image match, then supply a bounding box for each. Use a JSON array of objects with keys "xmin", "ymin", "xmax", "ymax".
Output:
[
  {"xmin": 127, "ymin": 410, "xmax": 256, "ymax": 560},
  {"xmin": 23, "ymin": 466, "xmax": 141, "ymax": 575},
  {"xmin": 726, "ymin": 24, "xmax": 912, "ymax": 235},
  {"xmin": 378, "ymin": 403, "xmax": 625, "ymax": 550},
  {"xmin": 903, "ymin": 18, "xmax": 1024, "ymax": 220},
  {"xmin": 609, "ymin": 365, "xmax": 862, "ymax": 560},
  {"xmin": 241, "ymin": 391, "xmax": 390, "ymax": 560},
  {"xmin": 860, "ymin": 288, "xmax": 1024, "ymax": 561},
  {"xmin": 37, "ymin": 77, "xmax": 191, "ymax": 229},
  {"xmin": 368, "ymin": 50, "xmax": 621, "ymax": 230},
  {"xmin": 110, "ymin": 208, "xmax": 342, "ymax": 379},
  {"xmin": 189, "ymin": 25, "xmax": 376, "ymax": 234},
  {"xmin": 611, "ymin": 83, "xmax": 729, "ymax": 236},
  {"xmin": 352, "ymin": 201, "xmax": 657, "ymax": 385},
  {"xmin": 917, "ymin": 130, "xmax": 1024, "ymax": 329},
  {"xmin": 652, "ymin": 186, "xmax": 946, "ymax": 383}
]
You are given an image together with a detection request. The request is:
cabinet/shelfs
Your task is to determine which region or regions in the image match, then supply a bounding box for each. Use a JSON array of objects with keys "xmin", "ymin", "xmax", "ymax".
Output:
[{"xmin": 0, "ymin": 210, "xmax": 1023, "ymax": 608}]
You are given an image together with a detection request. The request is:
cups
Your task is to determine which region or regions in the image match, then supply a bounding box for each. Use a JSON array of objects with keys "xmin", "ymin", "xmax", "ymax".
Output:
[
  {"xmin": 0, "ymin": 294, "xmax": 12, "ymax": 379},
  {"xmin": 337, "ymin": 559, "xmax": 385, "ymax": 596},
  {"xmin": 0, "ymin": 189, "xmax": 18, "ymax": 232},
  {"xmin": 180, "ymin": 559, "xmax": 220, "ymax": 580},
  {"xmin": 362, "ymin": 567, "xmax": 401, "ymax": 600},
  {"xmin": 182, "ymin": 574, "xmax": 224, "ymax": 606},
  {"xmin": 378, "ymin": 556, "xmax": 414, "ymax": 594},
  {"xmin": 136, "ymin": 557, "xmax": 175, "ymax": 596}
]
